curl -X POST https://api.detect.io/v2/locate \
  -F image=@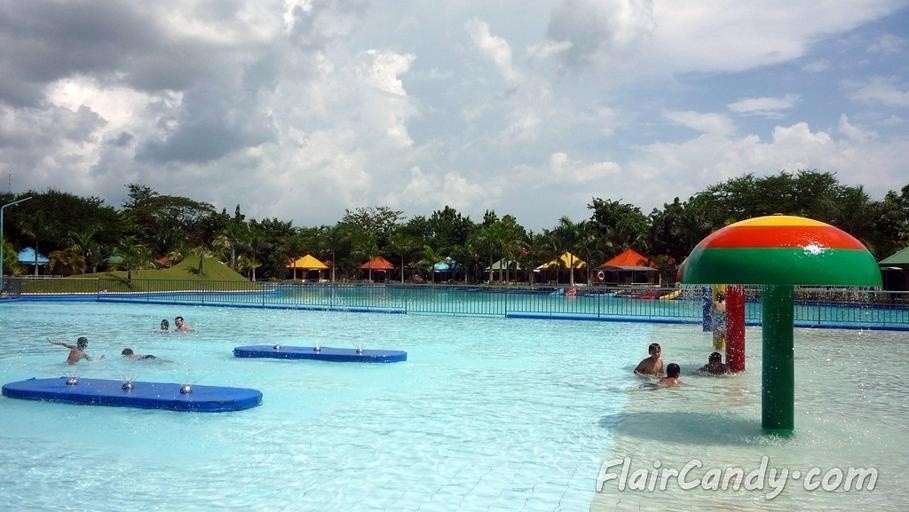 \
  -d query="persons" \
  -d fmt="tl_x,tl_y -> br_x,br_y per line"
693,352 -> 731,377
634,343 -> 665,388
608,362 -> 682,394
122,349 -> 175,364
157,319 -> 172,334
175,316 -> 200,334
709,290 -> 726,351
46,335 -> 106,366
